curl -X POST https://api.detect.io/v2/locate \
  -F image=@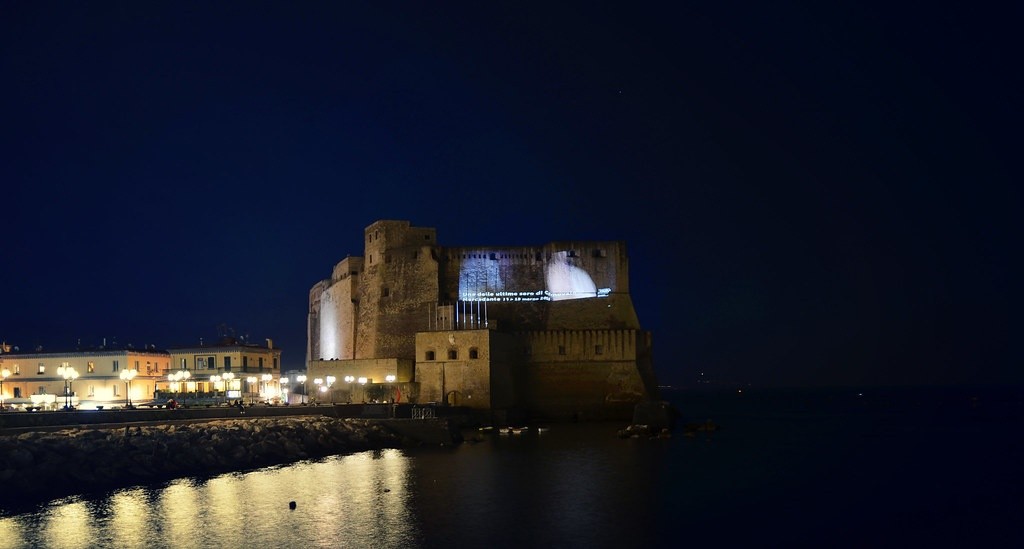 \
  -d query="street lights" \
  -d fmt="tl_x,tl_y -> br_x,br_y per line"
0,368 -> 11,409
167,371 -> 192,406
57,365 -> 80,409
209,371 -> 235,407
245,369 -> 396,404
119,368 -> 138,407
